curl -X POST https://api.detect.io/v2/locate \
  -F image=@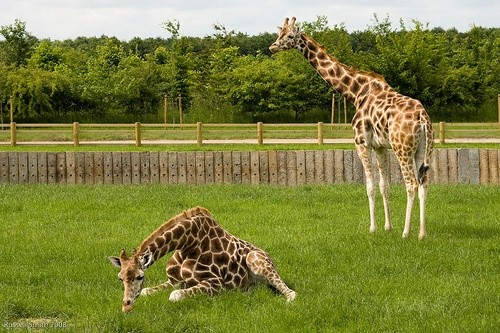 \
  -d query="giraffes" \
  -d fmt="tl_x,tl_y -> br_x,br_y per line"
107,205 -> 299,314
269,16 -> 435,242
